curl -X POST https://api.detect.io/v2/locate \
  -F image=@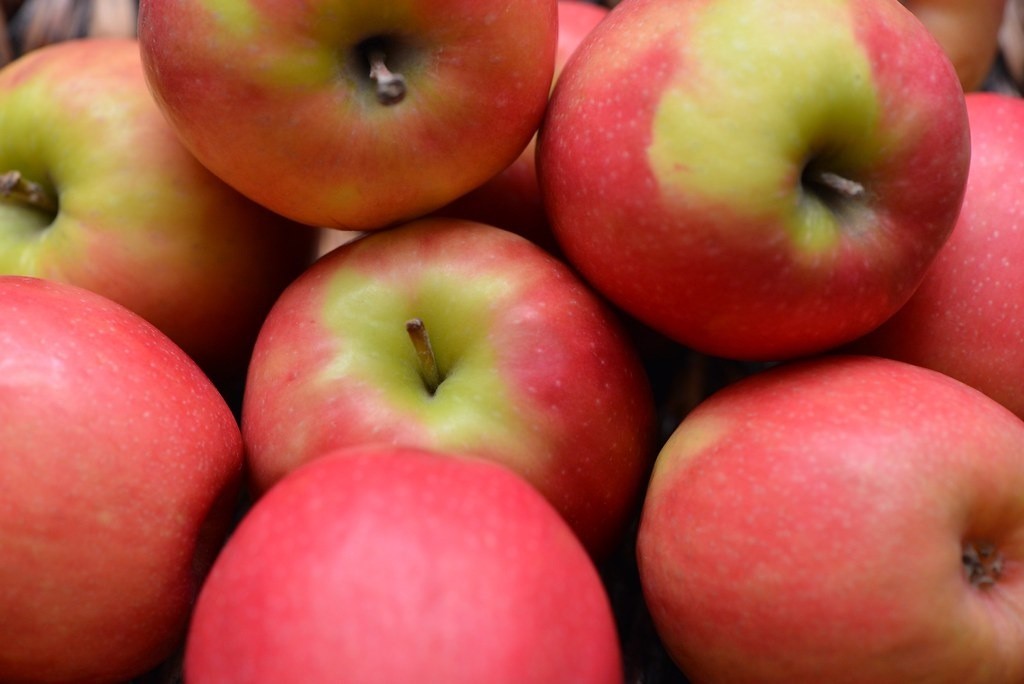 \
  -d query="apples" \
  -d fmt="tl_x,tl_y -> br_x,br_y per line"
0,0 -> 1024,684
